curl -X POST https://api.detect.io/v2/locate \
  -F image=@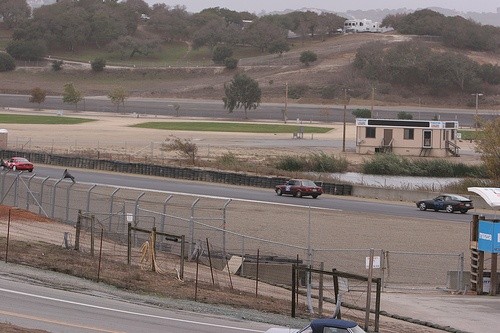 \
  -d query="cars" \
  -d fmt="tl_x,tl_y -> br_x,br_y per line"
416,194 -> 474,214
4,157 -> 34,172
264,319 -> 368,333
275,178 -> 323,198
